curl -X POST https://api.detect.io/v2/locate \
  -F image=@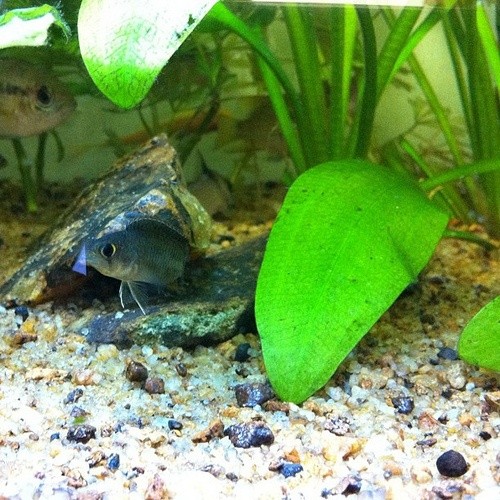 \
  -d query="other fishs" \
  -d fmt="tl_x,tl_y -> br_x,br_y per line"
0,58 -> 77,138
77,219 -> 191,315
